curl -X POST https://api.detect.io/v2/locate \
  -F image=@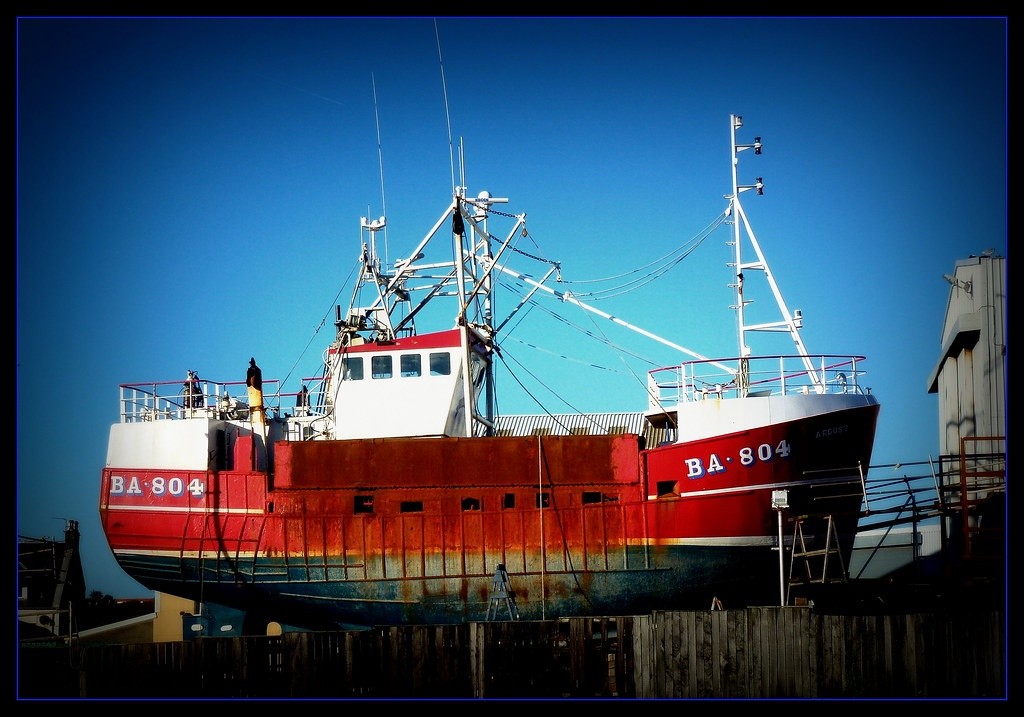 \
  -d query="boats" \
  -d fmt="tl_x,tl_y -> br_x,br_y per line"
99,21 -> 883,620
